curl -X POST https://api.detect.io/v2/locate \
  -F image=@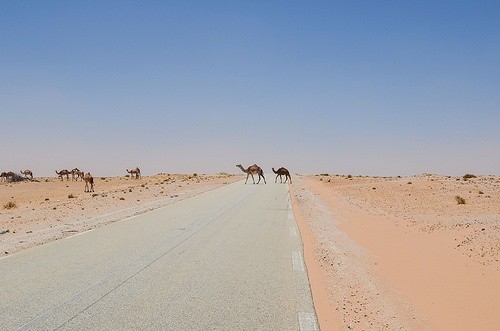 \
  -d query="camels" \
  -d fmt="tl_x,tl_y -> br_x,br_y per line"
235,163 -> 266,184
272,167 -> 292,184
72,170 -> 94,192
0,170 -> 33,182
126,165 -> 141,177
55,168 -> 84,182
